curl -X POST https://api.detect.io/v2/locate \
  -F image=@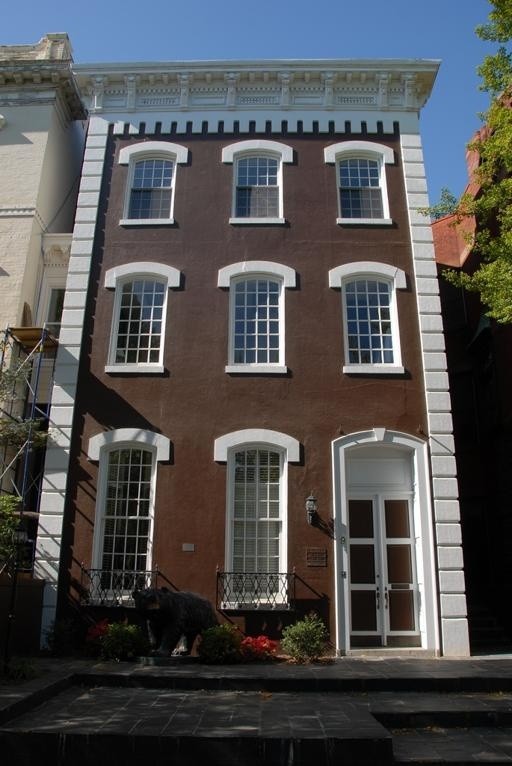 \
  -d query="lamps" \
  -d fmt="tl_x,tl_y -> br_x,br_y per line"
305,489 -> 315,523
